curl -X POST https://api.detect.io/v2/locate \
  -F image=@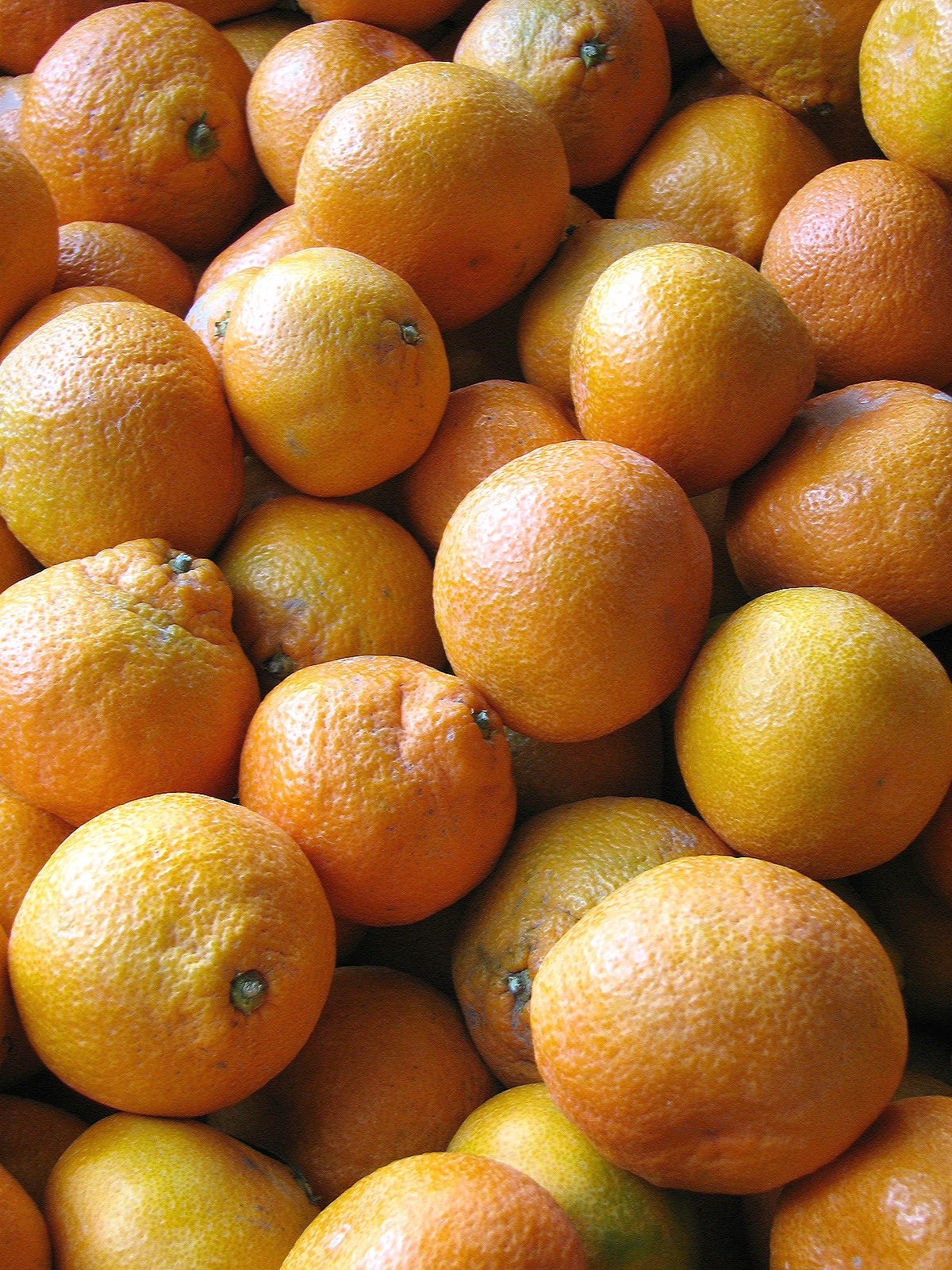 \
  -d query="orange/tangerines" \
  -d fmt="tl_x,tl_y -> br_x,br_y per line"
0,0 -> 952,1270
